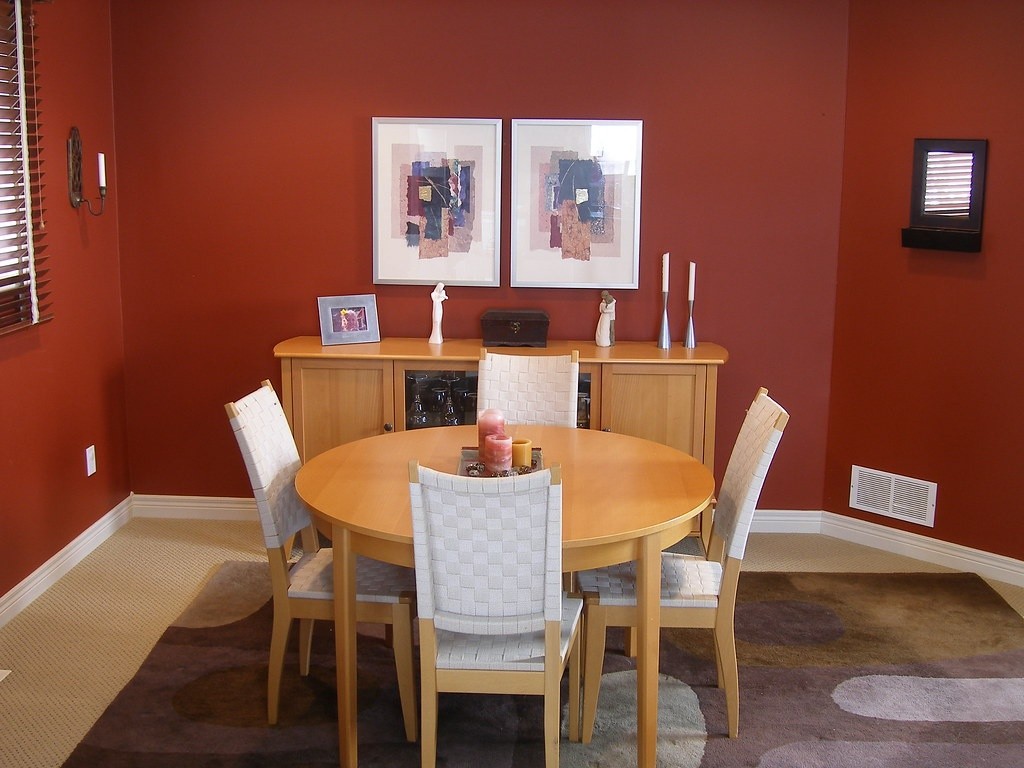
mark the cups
[405,372,476,429]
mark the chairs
[575,386,789,746]
[406,457,586,767]
[228,379,419,746]
[477,345,580,431]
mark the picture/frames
[510,120,646,289]
[371,117,502,288]
[316,294,380,345]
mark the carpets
[60,557,1024,768]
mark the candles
[662,252,669,292]
[689,261,696,301]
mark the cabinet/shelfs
[273,335,729,552]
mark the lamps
[69,128,107,215]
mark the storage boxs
[480,308,549,348]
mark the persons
[341,310,358,332]
[428,282,448,344]
[595,291,616,347]
